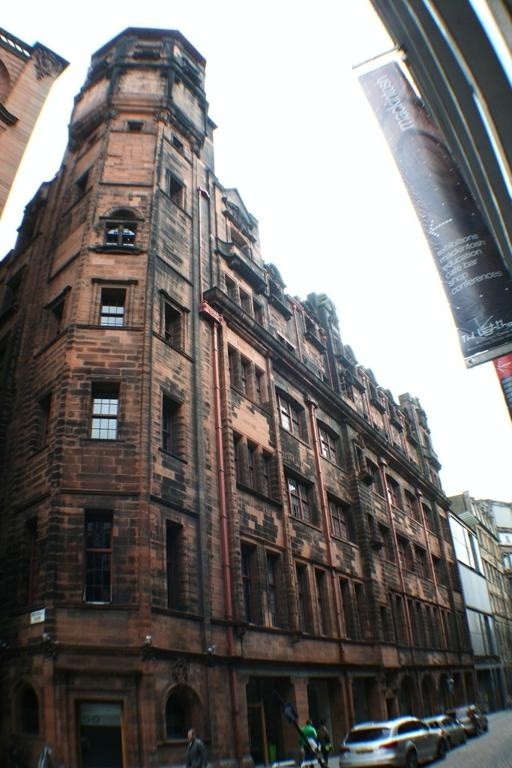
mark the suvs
[338,714,447,768]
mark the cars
[422,714,468,751]
[446,703,489,737]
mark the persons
[185,727,207,768]
[299,719,316,767]
[39,743,55,768]
[314,727,333,767]
